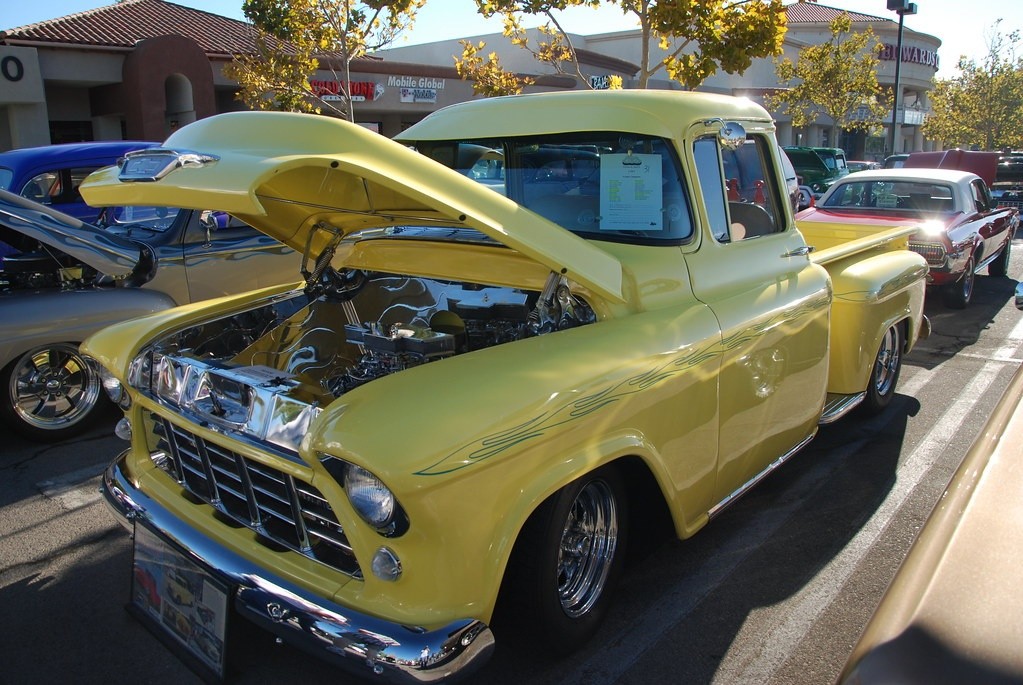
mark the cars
[794,149,1021,309]
[989,151,1023,230]
[458,143,611,200]
[781,145,910,210]
[0,141,232,276]
[1,187,396,440]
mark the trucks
[79,87,932,684]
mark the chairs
[660,199,772,238]
[529,193,618,233]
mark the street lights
[887,0,918,154]
